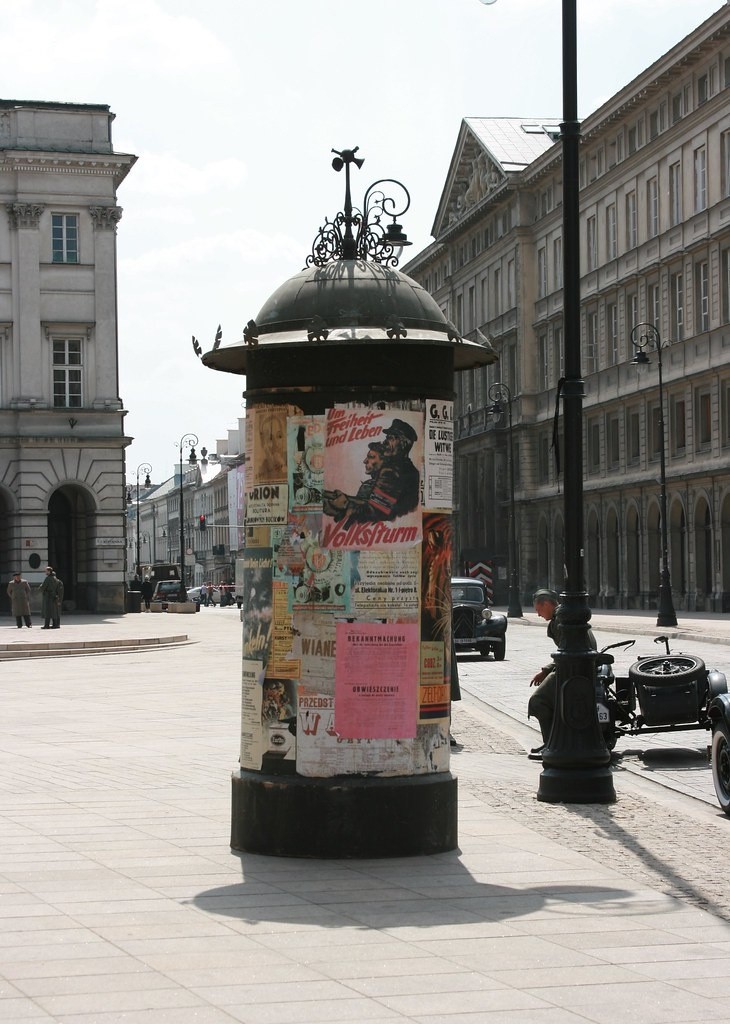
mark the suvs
[153,580,188,608]
[218,585,237,600]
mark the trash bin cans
[128,591,141,613]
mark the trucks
[135,561,183,596]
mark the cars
[186,586,221,604]
[451,578,507,661]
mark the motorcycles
[706,691,730,814]
[594,637,729,756]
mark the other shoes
[41,626,50,629]
[449,734,457,747]
[18,626,22,628]
[532,744,545,753]
[528,748,546,760]
[28,624,32,628]
[145,609,151,613]
[50,626,60,629]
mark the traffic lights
[200,514,207,531]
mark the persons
[198,581,216,607]
[37,566,64,629]
[7,572,32,628]
[528,590,597,761]
[218,581,231,606]
[130,574,153,613]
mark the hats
[13,573,21,576]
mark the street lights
[630,323,680,627]
[175,432,199,602]
[135,463,154,578]
[488,382,524,619]
